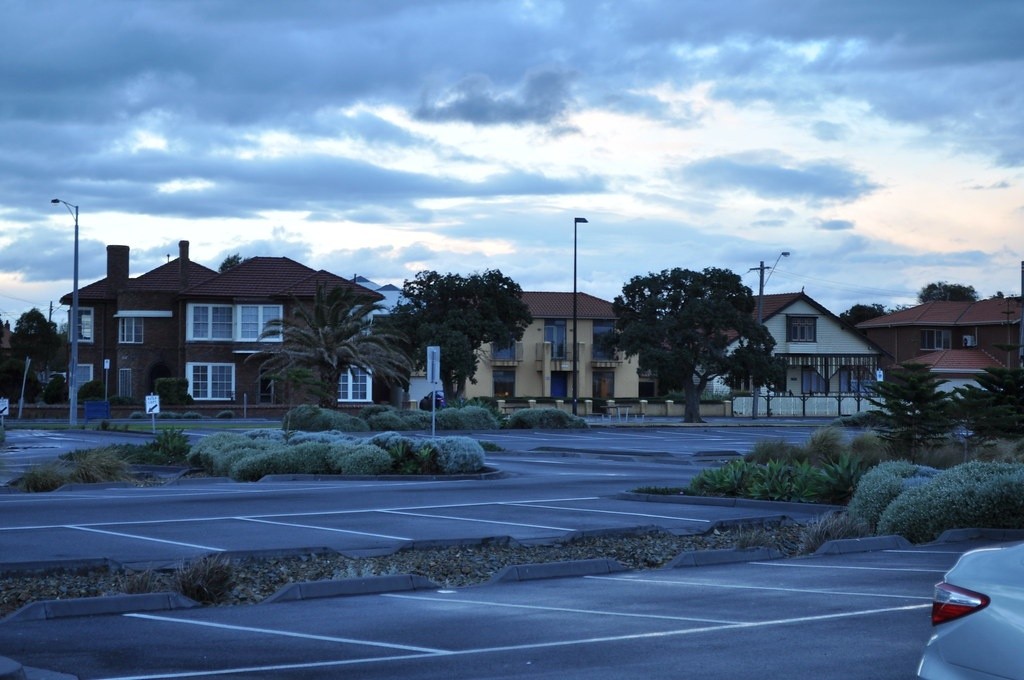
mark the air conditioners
[962,336,975,346]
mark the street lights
[569,217,589,416]
[50,198,81,425]
[752,251,790,419]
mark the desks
[600,405,633,421]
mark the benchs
[588,413,611,422]
[619,413,645,420]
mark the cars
[419,391,466,412]
[916,538,1024,680]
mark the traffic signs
[146,395,160,414]
[0,399,10,416]
